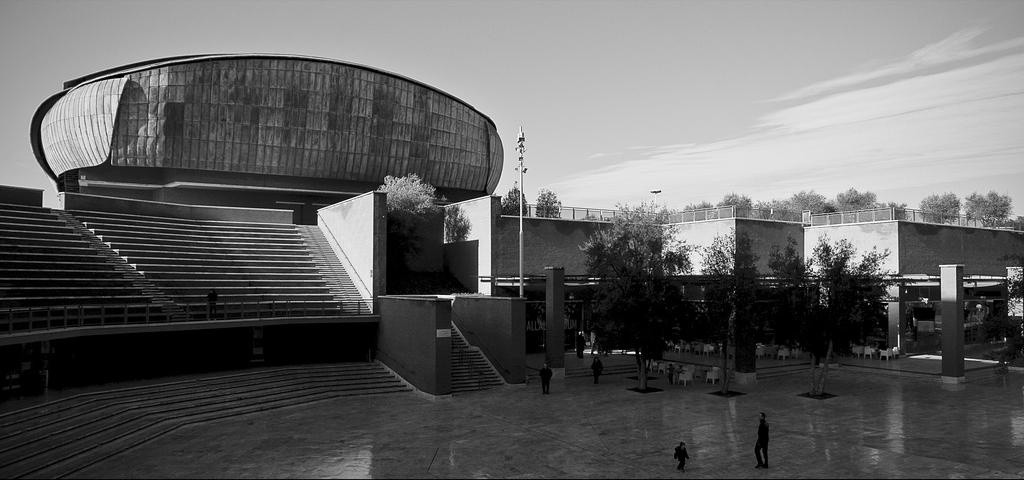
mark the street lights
[651,190,661,201]
[515,125,527,298]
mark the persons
[539,361,552,394]
[575,332,585,358]
[668,363,683,385]
[673,441,689,470]
[754,412,770,469]
[591,356,603,384]
[206,289,218,320]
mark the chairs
[651,359,720,387]
[665,339,802,361]
[852,343,900,360]
